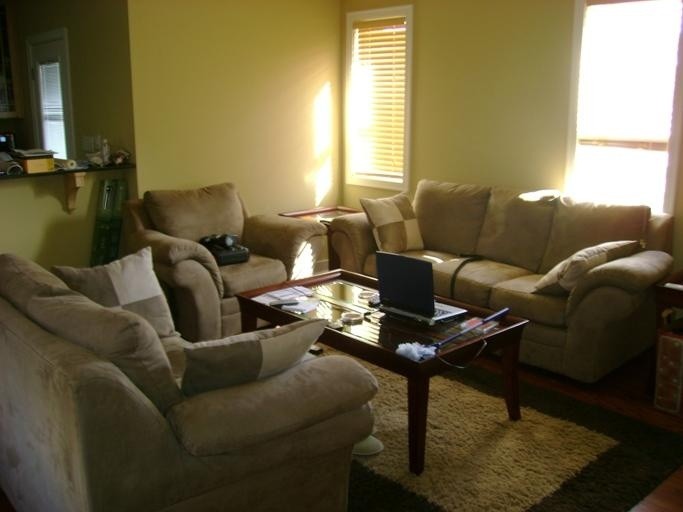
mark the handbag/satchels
[199,233,249,266]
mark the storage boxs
[12,152,53,175]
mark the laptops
[376,250,467,326]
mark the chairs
[124,182,328,343]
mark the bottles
[100,138,112,166]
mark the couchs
[0,244,378,512]
[327,179,675,384]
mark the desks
[277,205,363,229]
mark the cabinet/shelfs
[653,275,682,414]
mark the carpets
[306,341,683,511]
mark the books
[12,146,60,157]
[250,283,321,315]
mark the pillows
[528,239,640,296]
[360,191,425,253]
[51,244,178,340]
[174,314,327,395]
